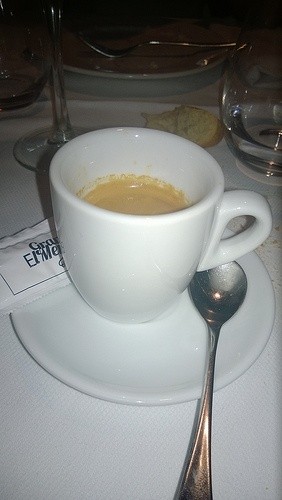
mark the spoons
[177,260,247,500]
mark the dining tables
[0,21,282,500]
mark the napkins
[0,211,72,315]
[238,27,282,90]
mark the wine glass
[14,1,93,173]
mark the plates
[59,15,233,78]
[9,227,276,407]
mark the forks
[75,30,238,57]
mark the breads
[141,105,224,147]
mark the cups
[48,126,273,325]
[0,0,54,113]
[218,0,282,186]
